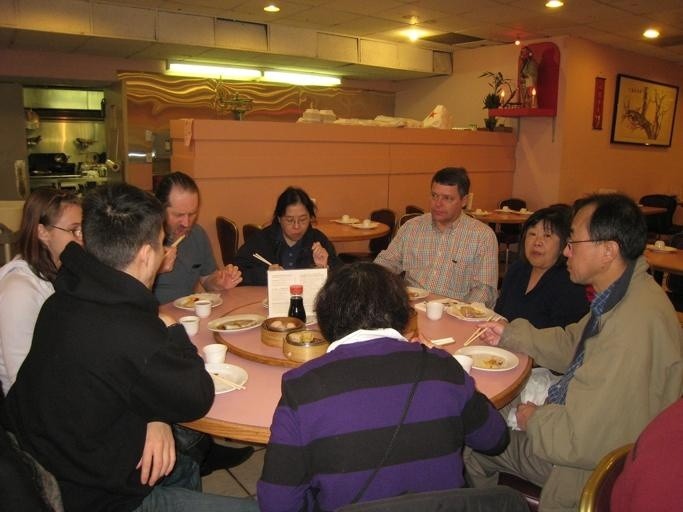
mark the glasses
[565,237,609,251]
[49,225,83,242]
[277,216,309,225]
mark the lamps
[162,57,342,88]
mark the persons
[461,193,683,512]
[493,207,590,331]
[371,167,499,310]
[154,171,245,305]
[234,187,339,285]
[256,261,511,511]
[609,395,683,512]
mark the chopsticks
[162,234,185,258]
[211,374,245,390]
[431,337,454,346]
[463,315,501,347]
[252,252,271,266]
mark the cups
[503,206,507,211]
[342,215,349,222]
[654,240,665,249]
[452,354,473,373]
[519,209,526,214]
[476,209,481,215]
[178,316,199,338]
[202,343,227,363]
[363,219,370,228]
[425,301,443,321]
[194,301,211,319]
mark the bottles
[288,285,305,323]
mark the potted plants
[477,70,513,131]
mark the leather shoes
[199,443,255,478]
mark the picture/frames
[610,74,678,148]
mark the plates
[471,212,492,217]
[203,363,248,394]
[206,313,266,332]
[646,246,676,254]
[352,224,377,230]
[447,304,494,322]
[407,287,428,300]
[495,210,514,214]
[335,219,358,224]
[454,345,520,371]
[514,212,534,215]
[173,292,222,311]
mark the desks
[636,241,682,310]
[465,208,536,283]
[259,215,389,257]
[633,203,666,238]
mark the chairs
[214,216,241,267]
[323,484,530,512]
[581,444,633,512]
[398,214,422,228]
[499,198,526,270]
[0,426,70,512]
[337,208,395,262]
[243,223,261,247]
[641,193,676,241]
[407,205,425,218]
